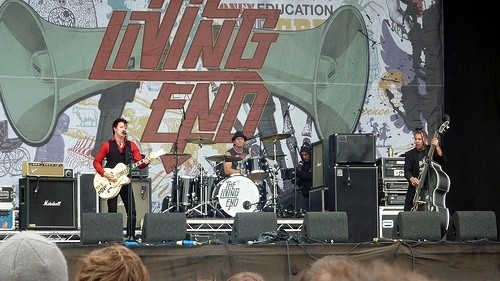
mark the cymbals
[260,132,291,143]
[164,152,191,157]
[183,137,217,144]
[266,153,287,158]
[207,154,242,162]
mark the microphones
[182,106,187,120]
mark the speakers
[302,212,349,243]
[81,213,123,243]
[447,211,497,241]
[234,212,276,242]
[395,212,441,241]
[142,213,187,241]
[20,177,77,230]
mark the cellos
[411,112,451,241]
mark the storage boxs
[0,202,16,231]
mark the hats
[231,131,247,142]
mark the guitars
[93,148,167,199]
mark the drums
[171,176,200,208]
[244,155,270,180]
[211,173,260,217]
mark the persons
[0,230,71,281]
[291,141,314,212]
[402,129,446,210]
[217,131,252,177]
[73,241,153,281]
[221,254,429,281]
[94,118,151,242]
